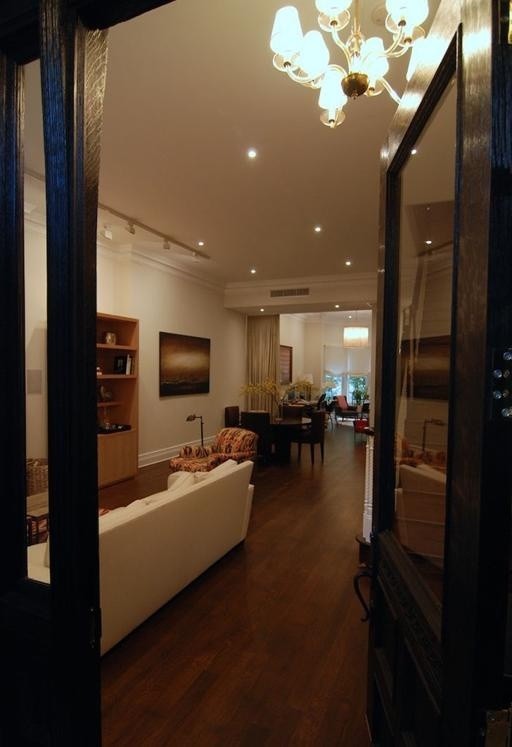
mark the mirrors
[393,71,445,608]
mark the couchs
[26,459,254,659]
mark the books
[113,354,133,374]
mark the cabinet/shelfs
[97,313,139,487]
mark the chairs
[396,463,446,562]
[225,405,325,469]
[169,427,259,479]
[333,395,358,422]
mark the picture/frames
[280,345,292,384]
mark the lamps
[270,0,428,129]
[344,310,369,348]
